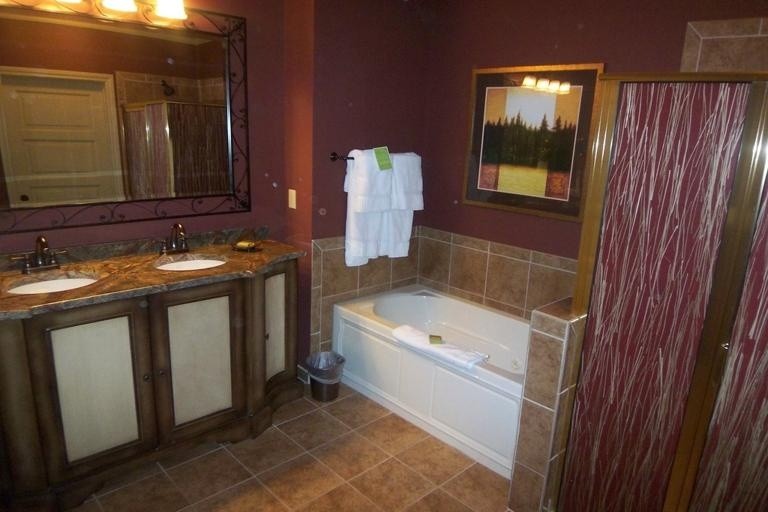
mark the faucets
[34,235,48,266]
[169,223,186,249]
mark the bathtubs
[330,282,531,481]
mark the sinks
[7,271,109,296]
[154,253,228,271]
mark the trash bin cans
[306,351,345,402]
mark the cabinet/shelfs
[249,258,304,440]
[0,280,250,512]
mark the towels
[391,322,490,370]
[344,148,424,268]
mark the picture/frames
[461,62,606,224]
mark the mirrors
[0,0,252,234]
